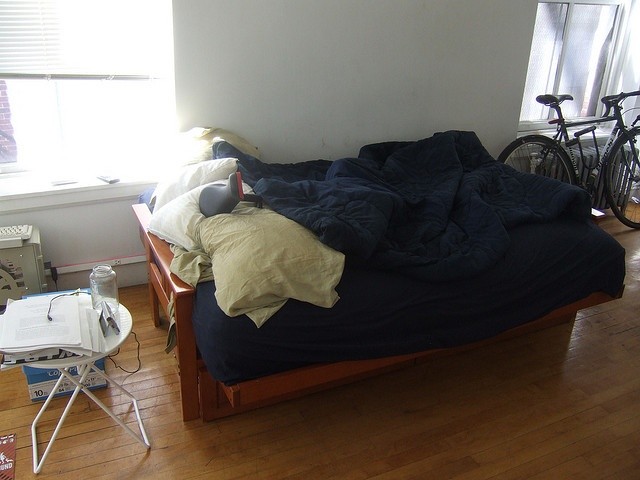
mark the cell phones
[99,300,120,338]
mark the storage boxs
[20,287,107,403]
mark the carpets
[0,432,16,480]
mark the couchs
[132,198,624,422]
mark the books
[0,288,107,357]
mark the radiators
[530,144,639,217]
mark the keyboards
[0,225,33,240]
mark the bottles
[89,265,119,315]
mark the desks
[6,292,151,475]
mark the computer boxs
[0,228,47,305]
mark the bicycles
[497,86,640,230]
